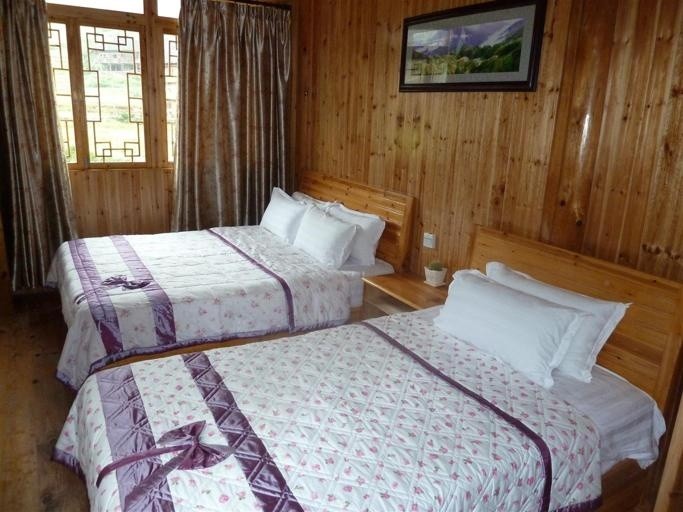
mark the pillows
[433,269,587,392]
[485,261,634,385]
[259,186,307,245]
[325,200,386,265]
[294,203,362,271]
[292,191,334,212]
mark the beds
[46,223,683,512]
[45,169,414,393]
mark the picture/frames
[399,0,548,93]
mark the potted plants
[423,260,448,288]
